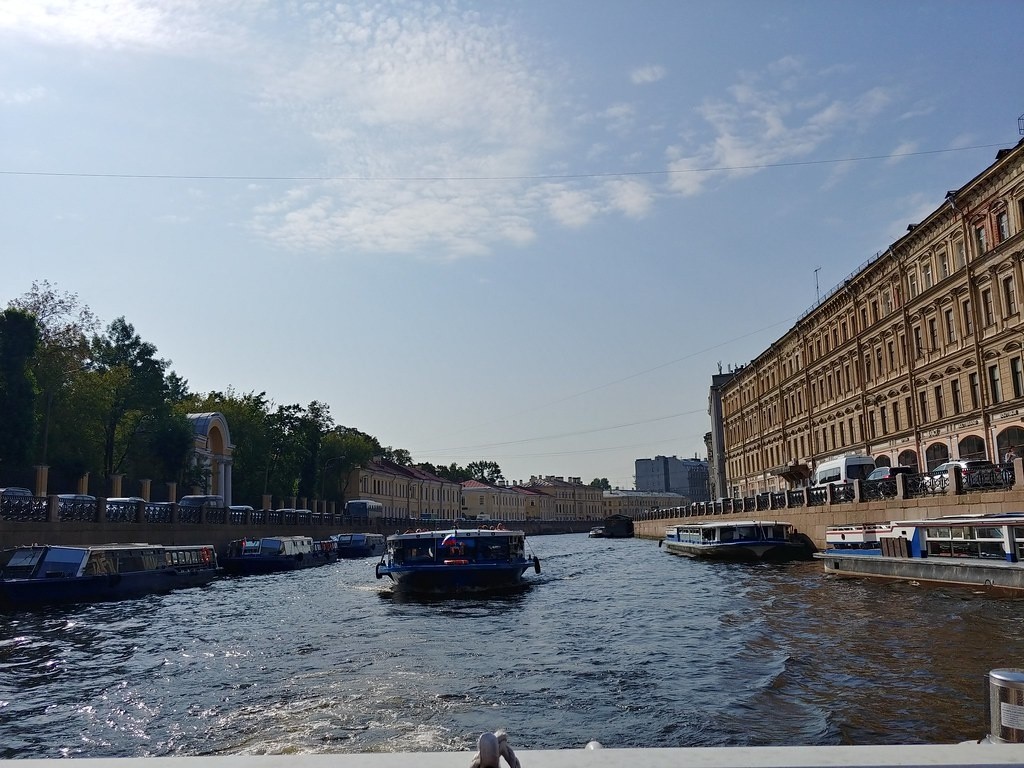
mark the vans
[180,493,224,509]
[811,455,876,498]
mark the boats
[226,534,338,573]
[374,521,540,592]
[328,530,385,558]
[812,511,1024,597]
[655,519,804,561]
[0,543,225,599]
[588,515,635,537]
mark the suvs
[862,465,917,498]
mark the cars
[107,498,144,515]
[228,504,254,522]
[919,458,1004,495]
[145,501,180,518]
[642,485,812,519]
[256,507,342,525]
[0,487,36,505]
[42,494,97,511]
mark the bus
[345,500,382,525]
